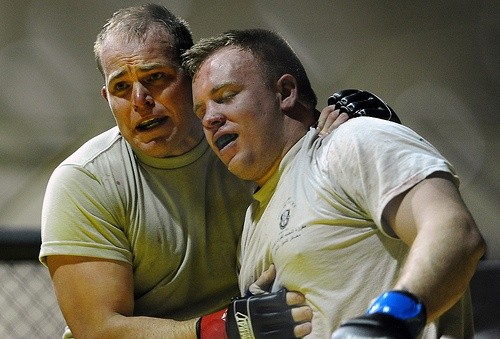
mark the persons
[181,29,486,339]
[39,3,401,339]
